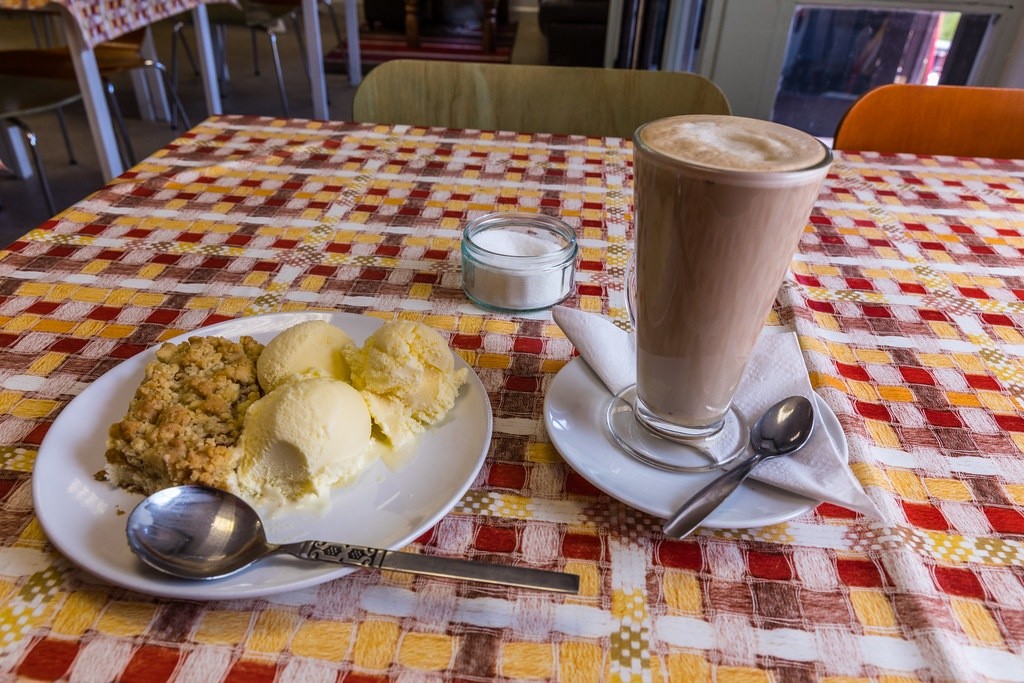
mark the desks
[0,116,1024,683]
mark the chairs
[832,84,1024,160]
[169,0,343,129]
[351,60,731,140]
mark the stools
[0,48,139,217]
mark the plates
[543,354,847,528]
[33,311,494,601]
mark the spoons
[662,395,814,540]
[127,484,580,594]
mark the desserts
[256,320,353,388]
[344,321,467,446]
[241,378,371,498]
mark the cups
[608,113,836,472]
[462,211,580,313]
